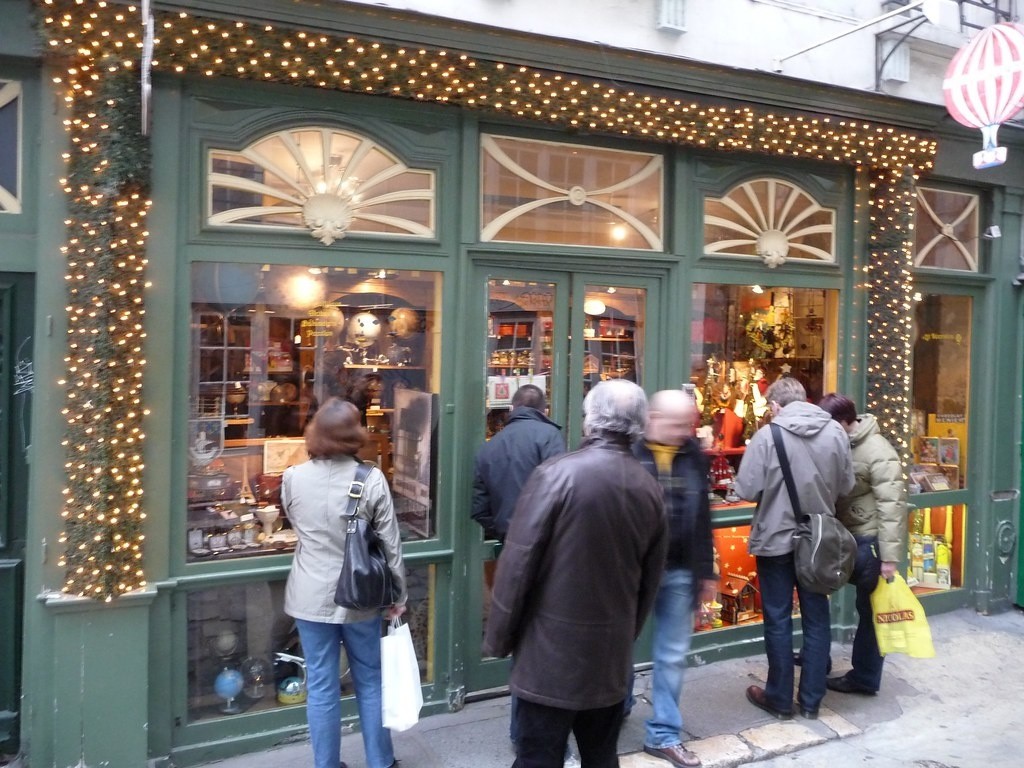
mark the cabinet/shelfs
[241,370,298,407]
[793,314,825,360]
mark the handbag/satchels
[333,461,399,609]
[795,509,858,595]
[870,571,937,659]
[380,615,424,732]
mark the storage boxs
[599,320,630,337]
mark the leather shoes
[643,743,702,768]
[793,652,832,674]
[825,670,875,696]
[799,706,818,719]
[747,685,791,719]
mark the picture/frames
[908,437,959,490]
[264,440,312,475]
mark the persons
[280,395,408,768]
[471,377,720,768]
[735,376,908,719]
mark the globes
[213,669,245,715]
